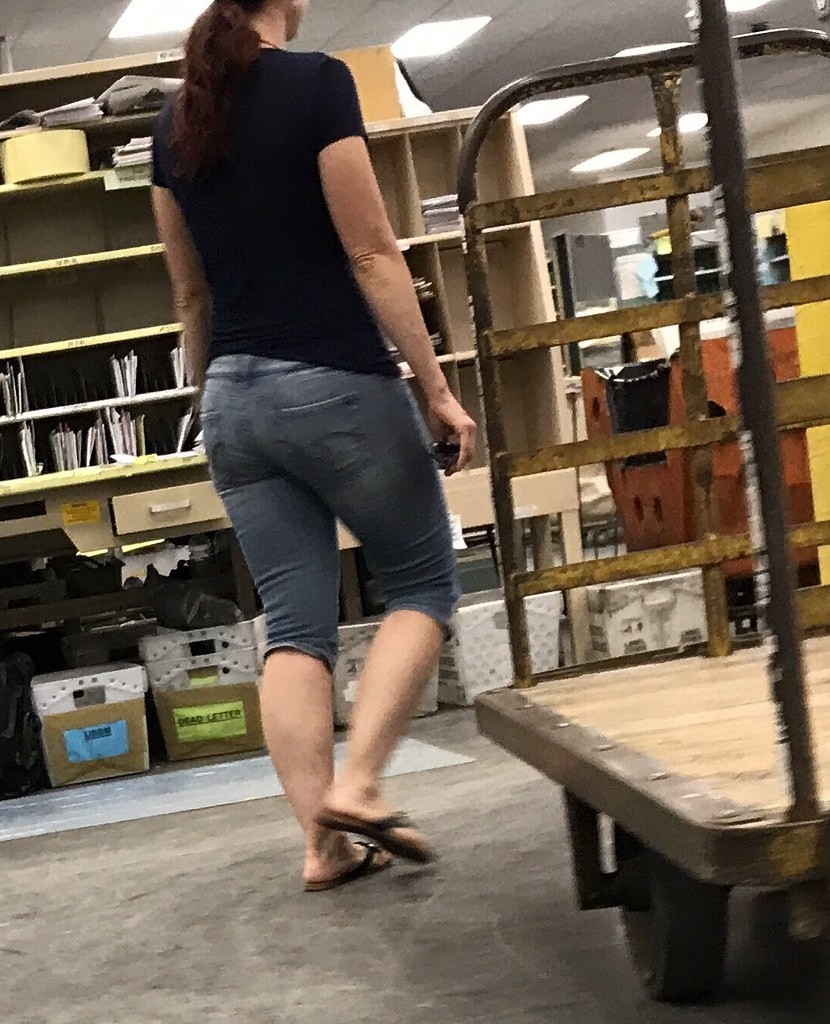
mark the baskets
[30,661,148,788]
[438,587,560,707]
[331,614,439,725]
[137,613,269,761]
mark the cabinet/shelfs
[368,102,575,449]
[0,45,208,496]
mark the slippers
[315,808,431,863]
[304,842,392,890]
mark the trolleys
[455,27,829,1006]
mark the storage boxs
[331,42,403,122]
[43,697,150,788]
[584,568,708,660]
[333,620,439,725]
[435,589,563,707]
[154,682,267,759]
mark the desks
[584,321,820,595]
[337,467,595,667]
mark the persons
[150,1,478,893]
